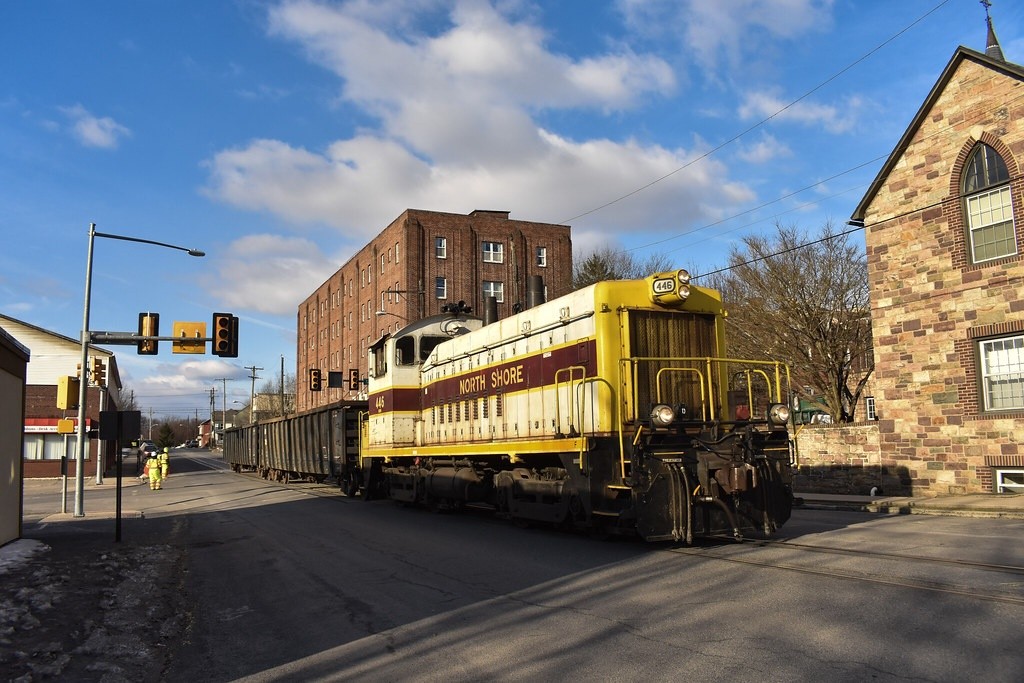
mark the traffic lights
[348,368,359,391]
[91,356,106,389]
[212,310,231,354]
[311,369,321,391]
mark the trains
[222,270,793,548]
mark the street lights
[233,400,253,424]
[150,424,158,440]
[74,221,205,518]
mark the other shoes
[152,487,156,489]
[156,488,163,489]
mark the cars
[143,445,159,459]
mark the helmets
[164,447,168,452]
[151,451,156,456]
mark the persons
[140,447,169,490]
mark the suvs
[189,441,198,448]
